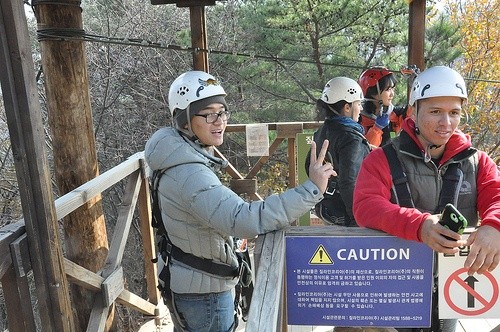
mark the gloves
[375,113,390,130]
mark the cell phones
[438,203,467,249]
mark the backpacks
[315,187,350,226]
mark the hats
[174,95,229,129]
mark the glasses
[195,111,231,124]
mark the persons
[351,66,500,276]
[144,70,338,332]
[357,66,416,152]
[305,76,373,227]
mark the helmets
[358,66,393,97]
[321,77,364,105]
[409,66,468,106]
[168,70,227,125]
